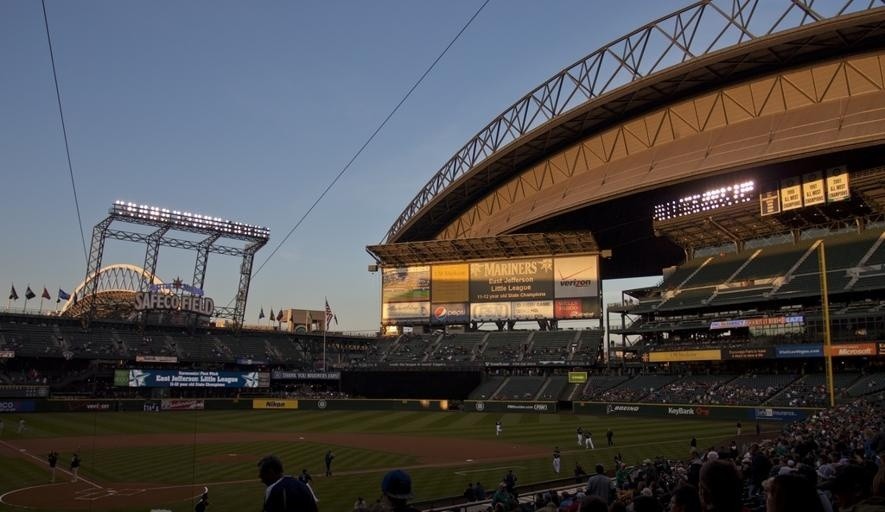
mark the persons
[1,318,885,512]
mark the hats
[816,461,872,491]
[379,468,417,501]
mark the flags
[9,284,19,300]
[43,287,50,299]
[259,309,283,320]
[326,301,332,330]
[25,286,35,300]
[60,289,70,301]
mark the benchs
[618,223,884,317]
